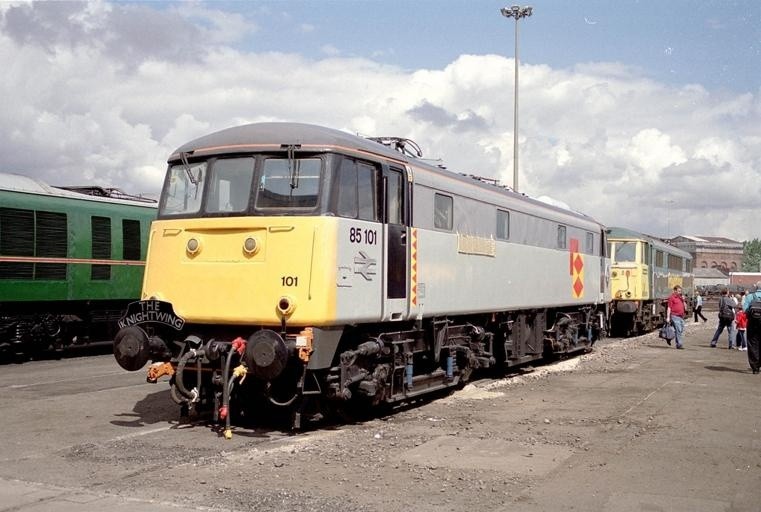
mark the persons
[711,280,761,374]
[665,285,688,349]
[694,291,708,322]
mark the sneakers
[710,343,716,348]
[729,345,747,351]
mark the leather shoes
[666,339,671,346]
[676,344,682,349]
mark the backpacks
[749,292,761,321]
[717,306,734,322]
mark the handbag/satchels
[659,324,675,339]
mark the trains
[112,123,694,442]
[1,170,165,364]
